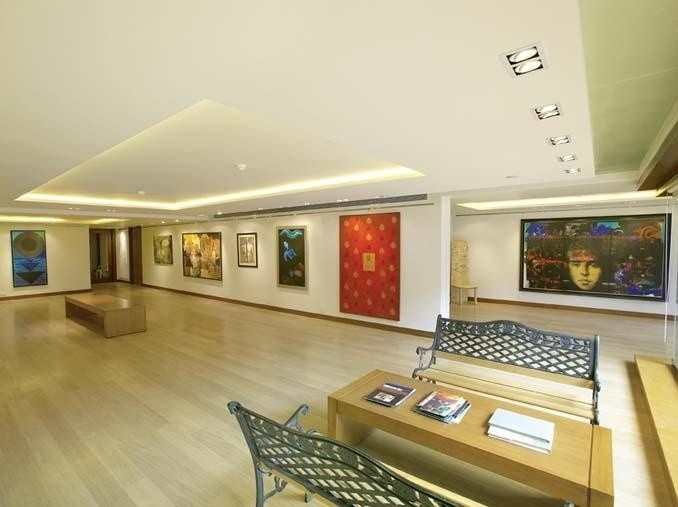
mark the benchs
[227,400,489,506]
[64,293,147,336]
[410,313,602,425]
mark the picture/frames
[518,212,671,302]
[274,224,309,289]
[237,232,258,267]
[181,231,222,281]
[10,230,48,287]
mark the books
[363,380,555,454]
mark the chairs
[96,264,109,279]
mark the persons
[282,239,298,279]
[558,238,615,294]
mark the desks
[452,283,479,304]
